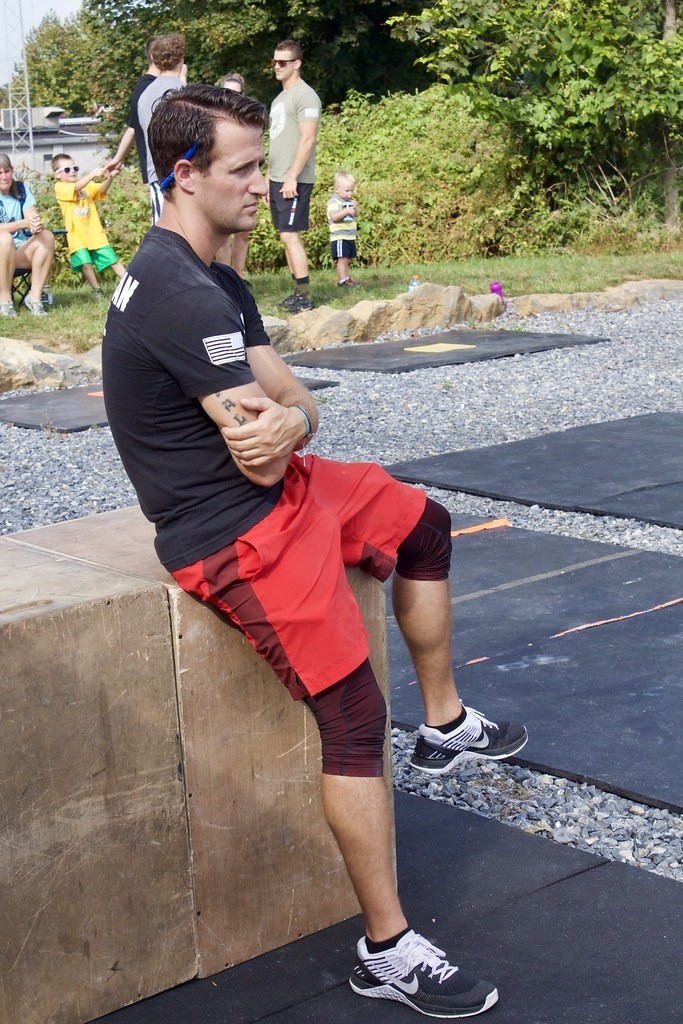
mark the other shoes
[93,288,104,298]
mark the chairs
[10,230,68,308]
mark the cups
[490,281,504,305]
[342,202,355,222]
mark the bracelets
[293,404,313,440]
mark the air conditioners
[0,109,29,130]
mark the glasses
[55,165,79,173]
[271,59,297,67]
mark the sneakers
[338,278,354,287]
[349,929,499,1018]
[24,290,48,316]
[273,291,316,314]
[409,699,528,774]
[0,299,17,317]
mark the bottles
[408,275,420,291]
[41,282,53,305]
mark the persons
[261,40,322,317]
[137,33,187,226]
[326,170,360,290]
[98,36,162,184]
[101,83,528,1018]
[0,153,54,318]
[216,72,249,279]
[51,153,126,300]
